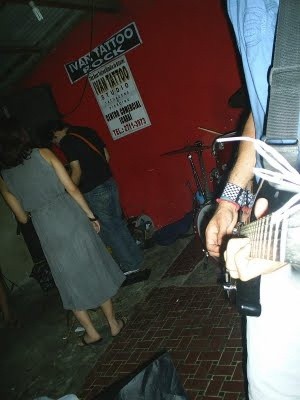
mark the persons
[48,125,144,275]
[205,0,300,400]
[0,282,13,321]
[0,120,129,347]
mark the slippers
[77,336,103,348]
[112,317,128,337]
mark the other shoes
[121,268,151,288]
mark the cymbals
[160,146,212,157]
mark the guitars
[217,178,300,317]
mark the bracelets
[222,182,245,203]
[89,215,98,221]
[215,195,241,210]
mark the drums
[193,199,220,267]
[213,132,241,175]
